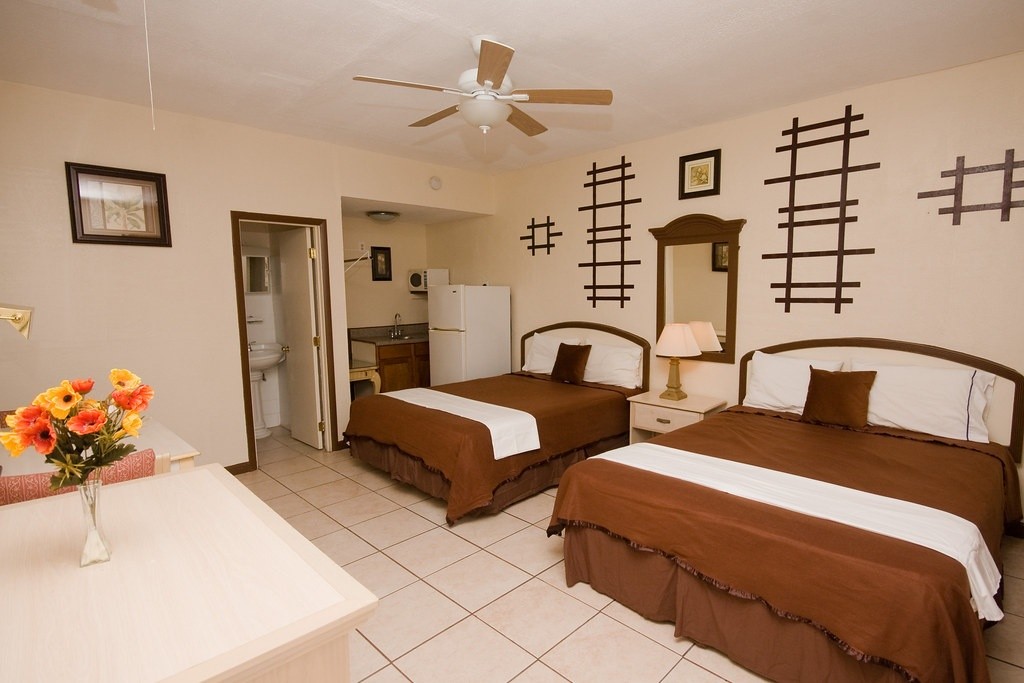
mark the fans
[352,34,614,137]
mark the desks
[0,412,204,478]
[349,359,382,395]
[0,463,377,683]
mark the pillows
[798,364,878,432]
[850,358,996,444]
[740,350,844,415]
[550,342,592,385]
[521,332,582,375]
[582,339,642,389]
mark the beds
[545,337,1024,683]
[342,321,651,529]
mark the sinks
[395,336,414,339]
[248,351,277,358]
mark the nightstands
[627,389,727,445]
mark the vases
[76,479,111,567]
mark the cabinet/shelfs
[376,341,431,394]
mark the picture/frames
[678,148,722,200]
[371,246,393,281]
[712,242,728,272]
[65,161,173,248]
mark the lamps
[652,323,702,402]
[367,211,400,222]
[687,321,723,352]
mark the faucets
[389,313,404,338]
[248,339,256,351]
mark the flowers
[0,368,155,527]
[458,94,513,134]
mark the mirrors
[649,214,747,364]
[242,255,270,294]
